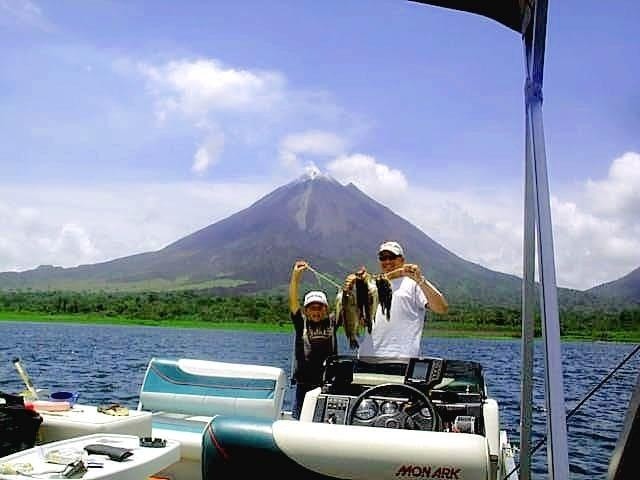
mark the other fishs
[335,266,392,349]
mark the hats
[380,241,406,258]
[304,290,330,304]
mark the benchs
[137,355,288,463]
[201,414,492,479]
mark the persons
[288,260,343,420]
[357,240,448,357]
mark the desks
[39,403,152,441]
[0,433,182,480]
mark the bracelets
[417,276,425,285]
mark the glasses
[379,254,401,261]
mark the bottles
[23,391,34,411]
